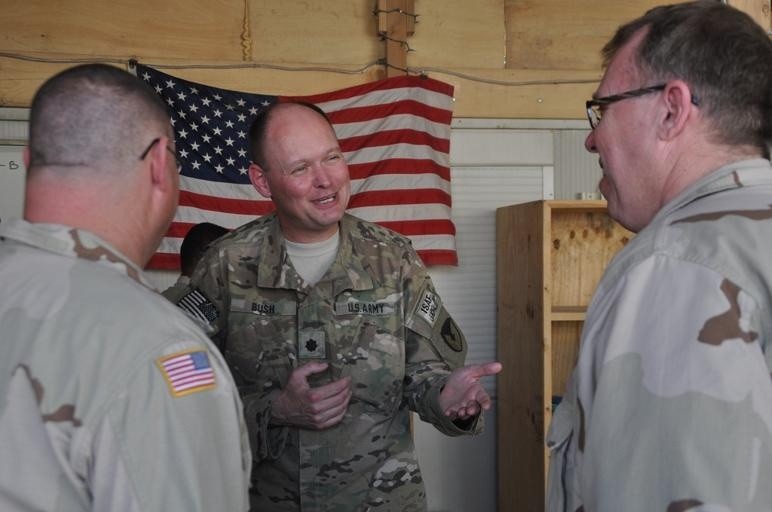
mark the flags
[126,53,461,273]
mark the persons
[170,100,503,512]
[542,0,770,512]
[1,61,255,512]
[157,219,237,305]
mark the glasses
[138,136,186,175]
[583,79,702,130]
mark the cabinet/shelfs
[495,198,640,511]
